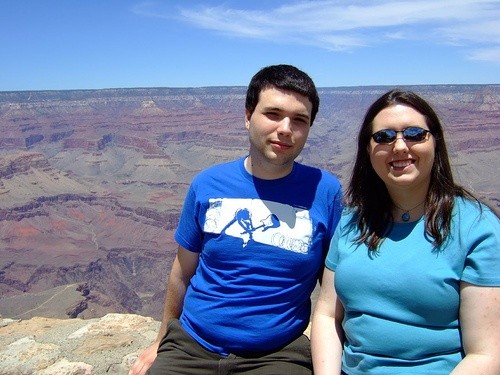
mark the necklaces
[394,199,427,222]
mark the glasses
[370,127,432,144]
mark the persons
[310,89,500,375]
[125,63,345,375]
